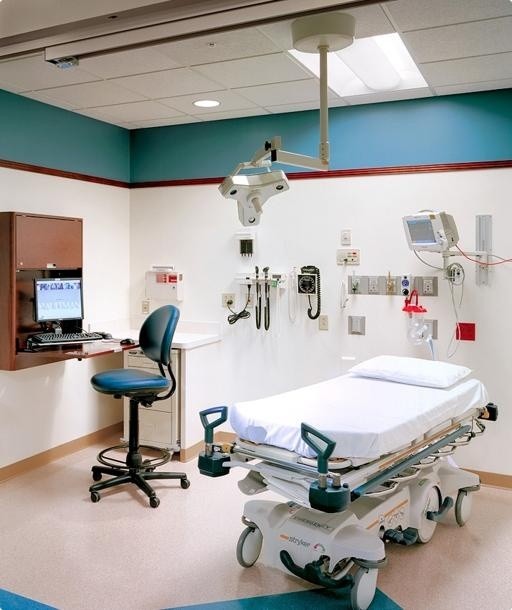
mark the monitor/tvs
[31,277,84,334]
[402,210,459,253]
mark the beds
[196,371,501,608]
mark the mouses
[120,338,135,345]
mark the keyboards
[32,332,103,343]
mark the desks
[120,336,221,464]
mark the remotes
[96,331,113,339]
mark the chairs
[88,304,190,510]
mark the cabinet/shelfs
[0,212,92,372]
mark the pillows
[349,355,473,389]
